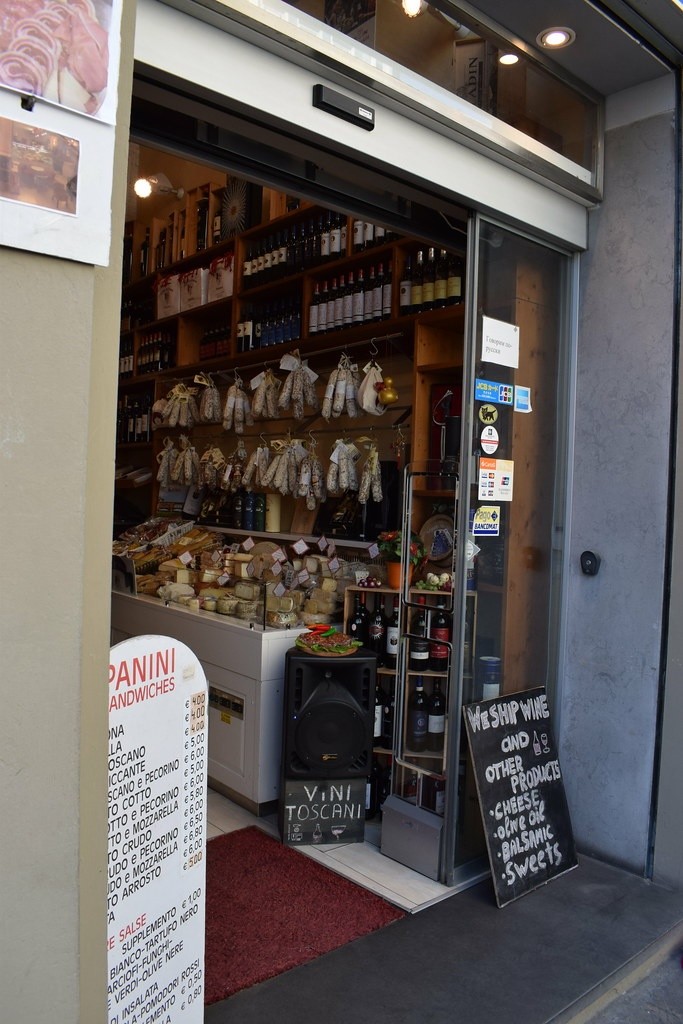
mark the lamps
[402,0,471,39]
[134,176,185,199]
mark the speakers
[281,646,377,779]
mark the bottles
[365,674,445,822]
[117,191,468,444]
[347,591,452,672]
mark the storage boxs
[157,256,234,320]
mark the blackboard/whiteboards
[463,686,580,909]
[285,779,367,845]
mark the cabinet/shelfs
[400,587,478,834]
[113,172,559,835]
[345,585,407,794]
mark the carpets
[204,825,406,1005]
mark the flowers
[377,529,430,576]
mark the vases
[386,561,413,590]
